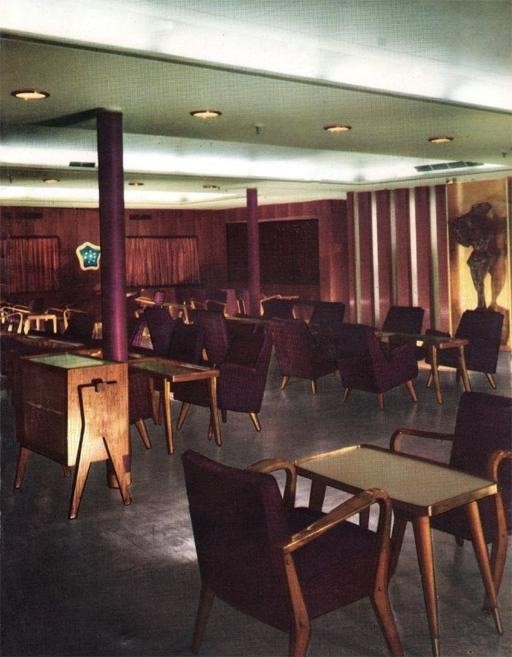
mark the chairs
[181,449,405,655]
[387,391,509,613]
[260,295,504,410]
[1,287,273,455]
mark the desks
[293,444,504,656]
[15,353,131,516]
